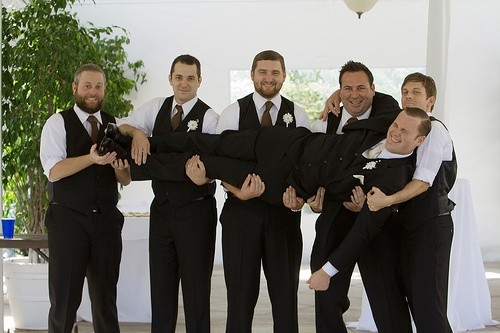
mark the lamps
[344,0,378,19]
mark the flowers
[186,119,199,132]
[283,113,293,128]
[362,160,382,170]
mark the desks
[0,235,78,333]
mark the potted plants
[0,0,148,330]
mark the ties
[87,115,99,143]
[261,101,276,126]
[365,142,385,158]
[171,105,185,129]
[347,117,359,124]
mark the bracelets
[290,209,301,212]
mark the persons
[310,60,414,333]
[114,55,221,333]
[215,50,310,333]
[98,107,431,290]
[39,63,135,333]
[319,73,457,333]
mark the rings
[187,165,191,167]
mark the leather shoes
[106,121,132,150]
[99,137,127,157]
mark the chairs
[78,180,155,322]
[346,178,500,333]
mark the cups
[1,218,16,238]
